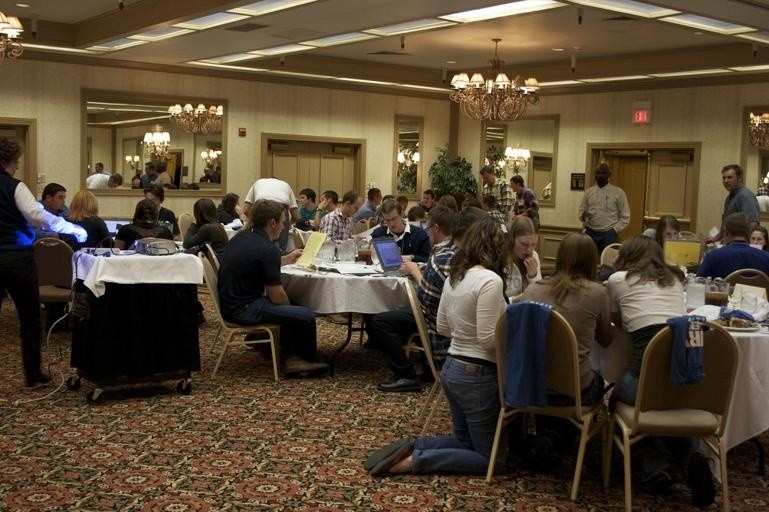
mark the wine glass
[728,286,742,311]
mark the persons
[480,166,515,214]
[578,164,630,254]
[31,182,244,259]
[87,162,221,190]
[0,133,87,389]
[510,175,541,230]
[365,214,769,507]
[703,164,760,244]
[217,177,504,391]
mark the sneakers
[693,459,717,508]
[365,437,406,469]
[371,442,413,478]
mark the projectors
[135,236,176,255]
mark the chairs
[484,301,603,502]
[400,331,451,362]
[197,251,284,382]
[36,238,81,339]
[177,212,376,275]
[403,276,446,443]
[599,242,624,266]
[603,318,741,511]
[724,267,767,289]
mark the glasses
[424,224,432,232]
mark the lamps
[200,147,222,169]
[446,39,542,124]
[501,141,530,173]
[745,108,768,149]
[124,151,140,170]
[167,101,224,137]
[140,117,172,155]
[0,13,30,61]
[397,142,420,169]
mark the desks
[677,311,769,460]
[278,257,409,377]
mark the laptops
[103,218,132,238]
[373,236,425,276]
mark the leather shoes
[284,356,330,378]
[379,376,420,393]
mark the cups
[740,295,757,316]
[356,238,372,265]
[695,276,727,293]
[339,239,354,261]
[401,255,411,264]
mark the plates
[712,320,761,331]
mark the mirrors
[478,113,560,209]
[738,104,768,224]
[392,112,425,202]
[78,87,230,199]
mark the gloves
[67,224,88,242]
